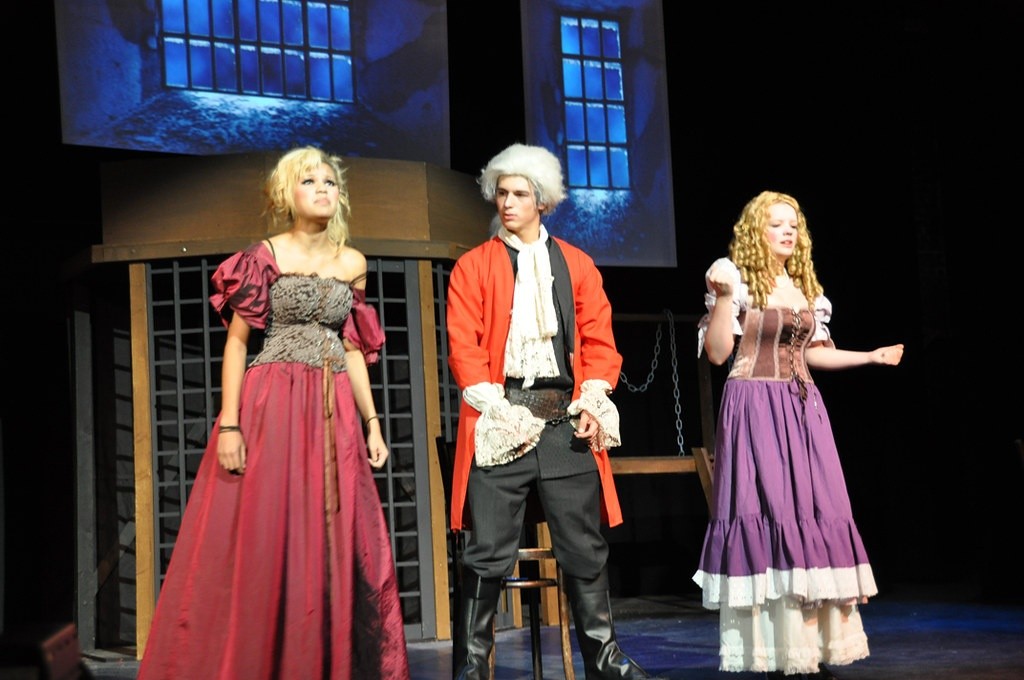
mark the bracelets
[219,426,242,433]
[365,416,379,428]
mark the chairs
[436,435,576,680]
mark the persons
[697,190,904,678]
[449,142,652,680]
[137,146,410,680]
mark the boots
[563,564,654,680]
[452,568,503,680]
